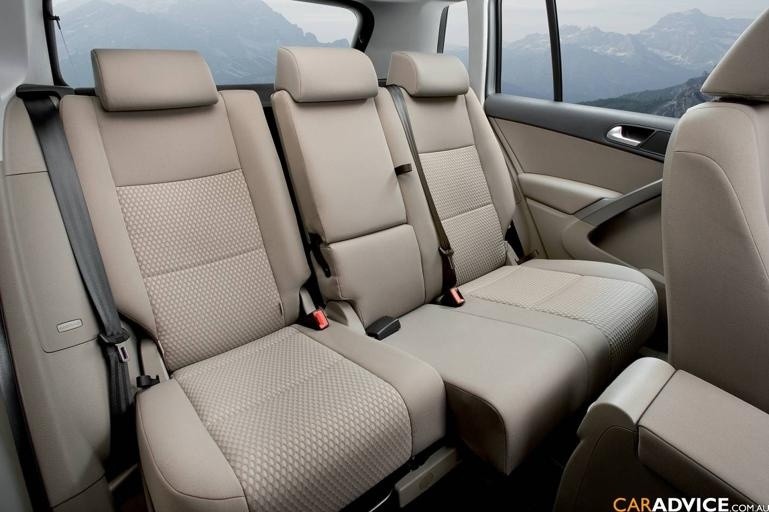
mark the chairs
[61,8,769,512]
